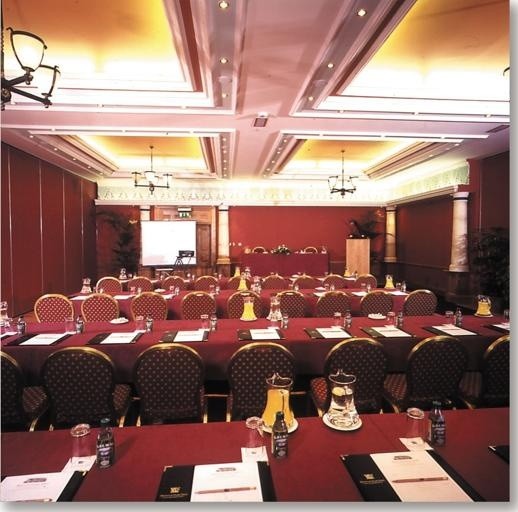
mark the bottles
[175,285,179,296]
[96,419,114,470]
[344,310,352,329]
[456,306,462,326]
[77,316,84,332]
[396,282,400,288]
[401,280,406,293]
[137,288,141,294]
[5,322,11,333]
[271,412,289,458]
[17,315,25,335]
[210,312,217,331]
[367,282,371,292]
[0,320,6,345]
[397,310,403,329]
[428,401,446,446]
[215,287,220,295]
[283,311,288,329]
[147,313,154,332]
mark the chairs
[292,275,317,290]
[403,289,437,316]
[81,293,120,322]
[227,290,263,320]
[314,291,353,318]
[359,290,394,317]
[280,290,308,319]
[307,337,388,416]
[0,349,49,433]
[260,274,287,289]
[161,275,187,292]
[34,293,75,323]
[95,276,123,294]
[351,273,378,289]
[180,291,216,321]
[461,337,510,407]
[382,334,468,413]
[321,274,347,289]
[130,342,208,427]
[194,275,217,291]
[129,292,168,320]
[303,245,318,253]
[126,276,154,292]
[40,345,130,430]
[226,341,297,423]
[226,276,251,291]
[251,246,266,254]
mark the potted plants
[460,223,510,313]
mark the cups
[69,423,93,467]
[332,312,341,330]
[384,274,396,290]
[241,296,257,320]
[130,286,136,294]
[288,283,294,290]
[445,311,455,328]
[405,408,426,450]
[503,310,509,325]
[135,313,145,334]
[386,312,397,330]
[63,315,76,334]
[233,266,241,278]
[200,314,209,331]
[209,284,214,292]
[396,283,401,291]
[360,282,366,292]
[267,296,283,320]
[237,275,249,291]
[81,278,92,294]
[0,300,11,328]
[261,372,295,429]
[244,417,265,461]
[327,368,360,429]
[170,285,174,294]
[269,313,279,329]
[324,283,329,292]
[476,294,492,316]
[343,265,351,277]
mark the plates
[322,413,364,432]
[473,313,494,318]
[259,418,299,434]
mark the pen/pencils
[442,329,460,330]
[110,335,134,338]
[392,477,448,483]
[178,333,202,336]
[254,332,274,334]
[15,499,51,502]
[194,487,256,494]
[379,330,398,332]
[35,336,54,339]
[322,330,341,332]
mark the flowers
[269,243,293,256]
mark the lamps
[0,18,65,112]
[130,173,173,197]
[327,172,360,198]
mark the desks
[240,254,329,279]
[67,289,410,316]
[0,404,509,503]
[0,313,511,380]
[119,276,357,291]
